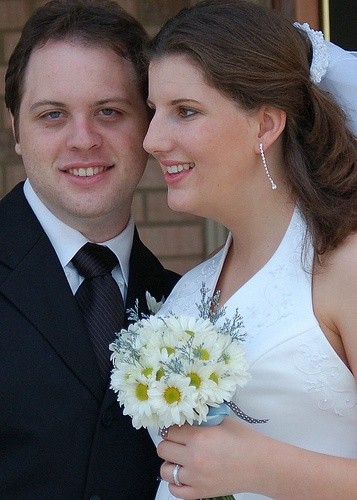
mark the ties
[70,243,126,392]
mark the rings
[172,465,182,487]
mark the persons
[0,2,185,500]
[143,3,355,500]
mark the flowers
[108,282,270,500]
